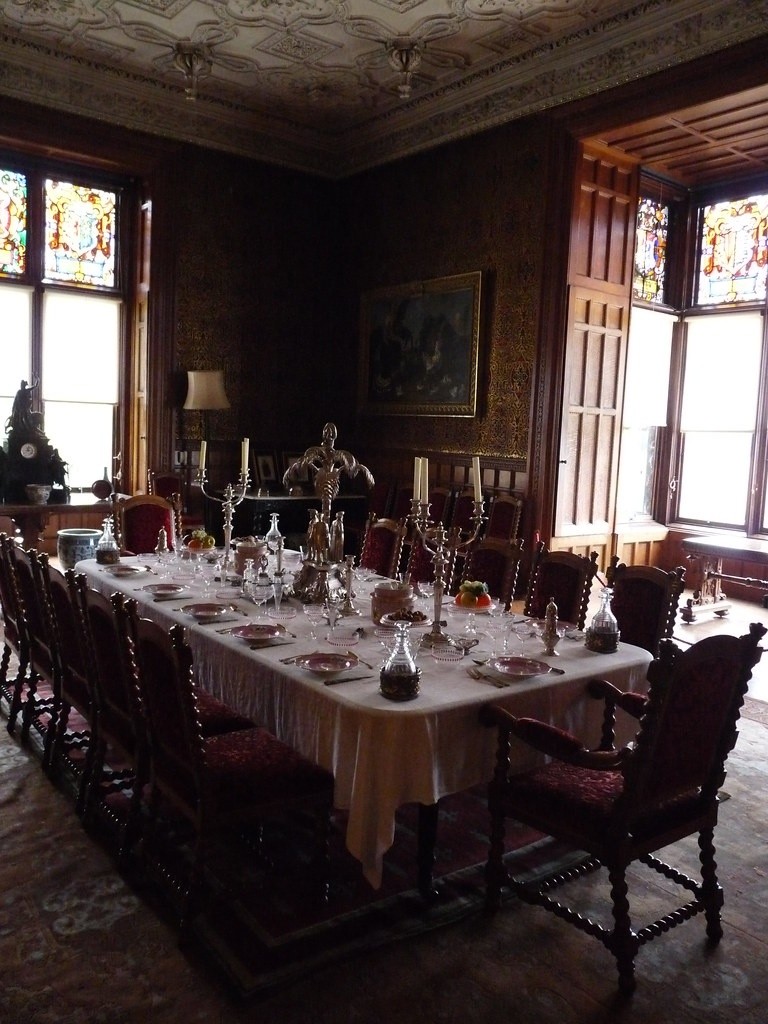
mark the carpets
[0,650,730,1014]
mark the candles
[413,456,421,500]
[421,456,429,504]
[472,457,482,502]
[243,438,249,474]
[241,441,245,474]
[199,441,207,469]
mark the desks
[210,486,368,549]
[45,544,656,904]
[0,492,133,555]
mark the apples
[182,534,215,548]
[455,591,491,606]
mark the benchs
[678,534,768,624]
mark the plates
[104,564,139,578]
[181,602,227,619]
[293,651,358,676]
[142,583,184,597]
[229,624,287,643]
[488,657,551,678]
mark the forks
[465,667,511,688]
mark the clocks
[20,442,38,460]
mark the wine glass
[151,536,527,671]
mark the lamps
[183,369,231,536]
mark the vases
[56,528,103,570]
[25,483,52,505]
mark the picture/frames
[282,450,315,487]
[251,448,281,489]
[355,269,485,418]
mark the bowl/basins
[56,528,102,569]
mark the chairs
[0,469,768,1000]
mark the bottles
[584,586,620,653]
[378,619,421,700]
[95,513,121,564]
